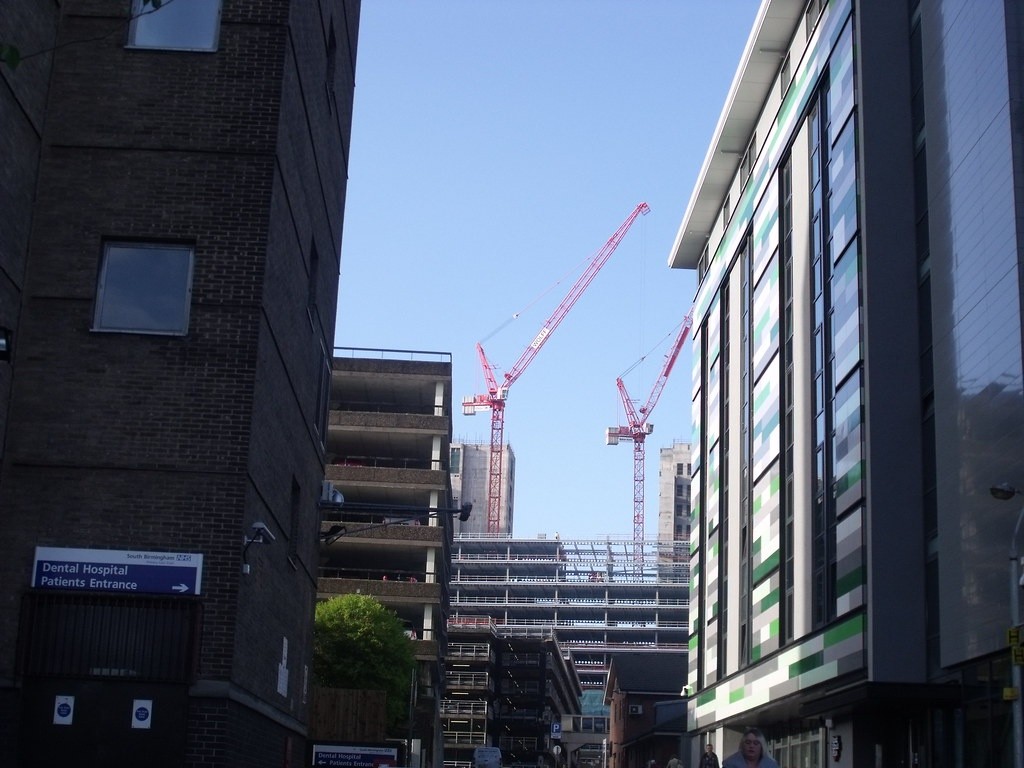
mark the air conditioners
[629,705,642,714]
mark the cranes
[461,202,650,533]
[606,306,694,583]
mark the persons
[666,754,685,768]
[699,744,719,768]
[722,729,779,768]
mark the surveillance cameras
[244,522,276,546]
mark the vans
[471,746,503,768]
